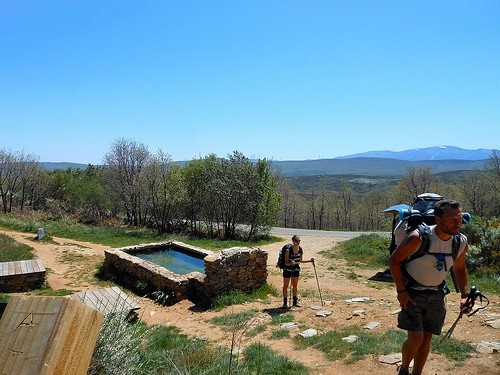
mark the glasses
[294,239,300,242]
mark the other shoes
[283,304,291,309]
[397,365,409,375]
[292,303,302,307]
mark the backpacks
[382,192,471,265]
[276,244,303,269]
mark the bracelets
[397,289,406,293]
[461,292,469,298]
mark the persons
[283,235,315,308]
[388,201,472,375]
